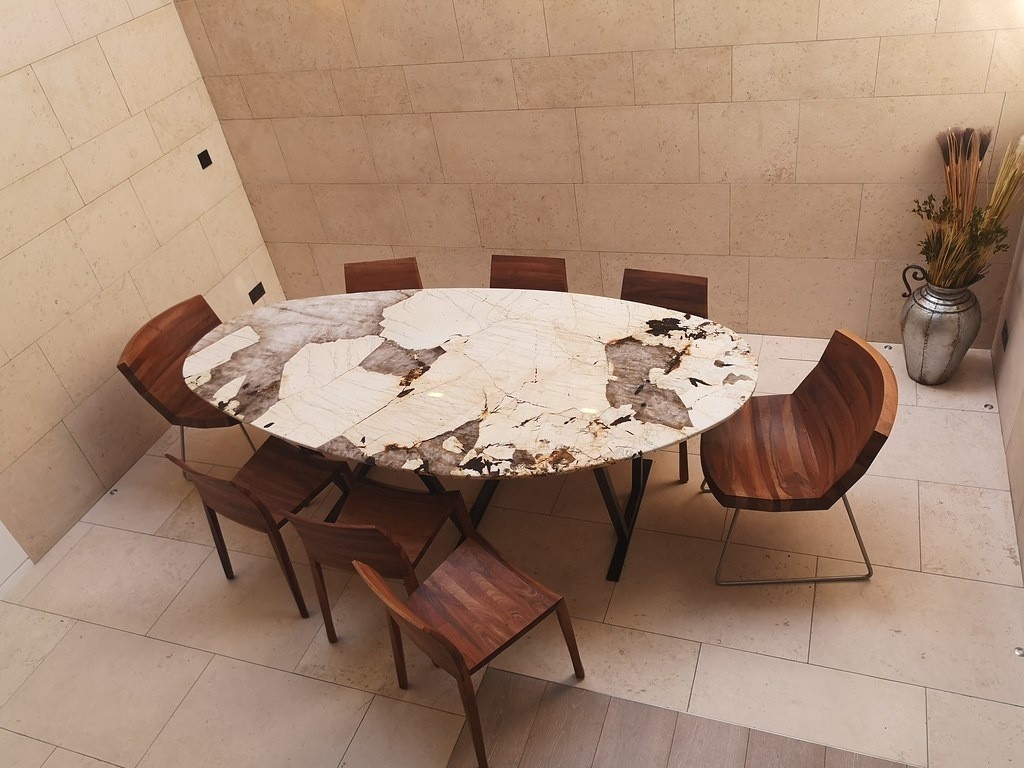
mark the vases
[901,265,982,385]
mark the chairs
[351,532,586,768]
[166,436,351,617]
[699,329,899,586]
[490,255,569,292]
[116,294,256,477]
[344,257,423,293]
[279,476,474,643]
[620,269,708,483]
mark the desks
[183,287,760,581]
